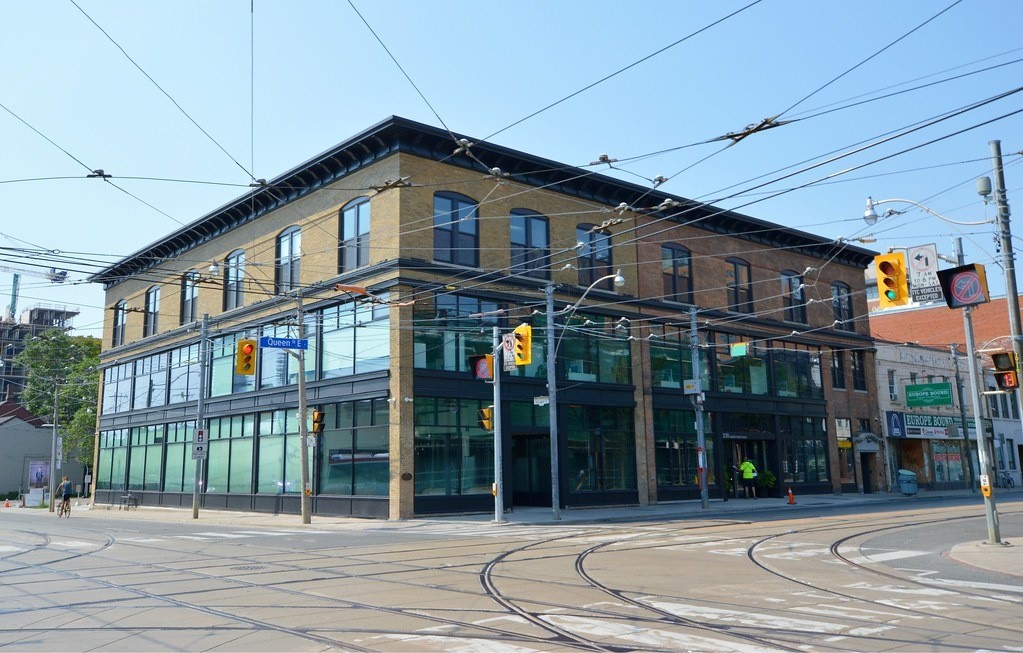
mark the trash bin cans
[899,470,918,495]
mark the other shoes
[754,496,757,500]
[743,495,749,499]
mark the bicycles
[56,493,71,518]
[998,470,1014,488]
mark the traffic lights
[478,409,491,432]
[313,410,325,434]
[237,339,255,375]
[514,325,532,366]
[935,263,991,310]
[990,354,1020,390]
[873,251,909,309]
[468,354,493,381]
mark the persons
[736,457,758,500]
[56,476,74,516]
[35,468,43,488]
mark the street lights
[208,258,311,524]
[862,139,1022,419]
[544,268,627,519]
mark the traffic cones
[5,498,9,507]
[787,486,797,504]
